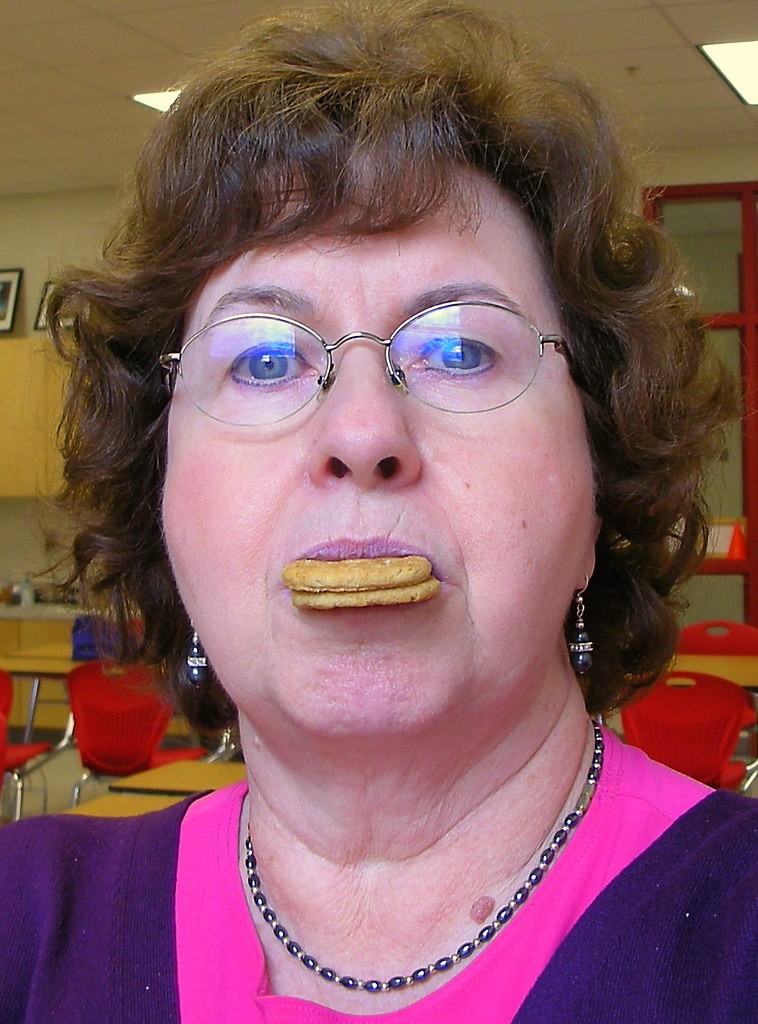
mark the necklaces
[245,718,605,993]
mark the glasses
[159,301,580,426]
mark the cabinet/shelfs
[0,338,89,499]
[0,619,194,738]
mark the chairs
[621,620,758,799]
[0,615,208,821]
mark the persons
[0,2,758,1024]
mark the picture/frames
[34,281,93,330]
[0,268,23,333]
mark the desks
[0,658,185,743]
[64,793,189,817]
[108,760,247,794]
[8,642,71,659]
[622,654,758,695]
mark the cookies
[282,555,432,590]
[291,579,438,609]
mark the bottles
[20,578,34,607]
